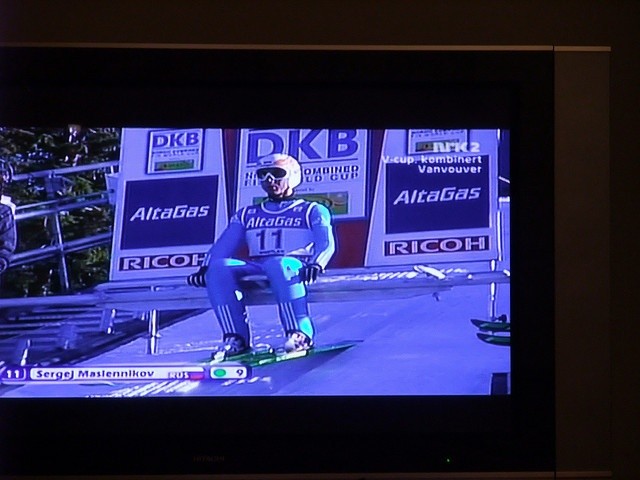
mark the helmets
[256,153,302,188]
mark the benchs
[94,270,510,358]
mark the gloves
[301,264,323,285]
[187,266,208,287]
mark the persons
[0,160,17,277]
[187,154,336,363]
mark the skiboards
[196,339,363,367]
[471,319,511,346]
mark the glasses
[256,167,287,182]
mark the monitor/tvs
[0,40,614,475]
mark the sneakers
[210,336,246,359]
[283,331,310,353]
